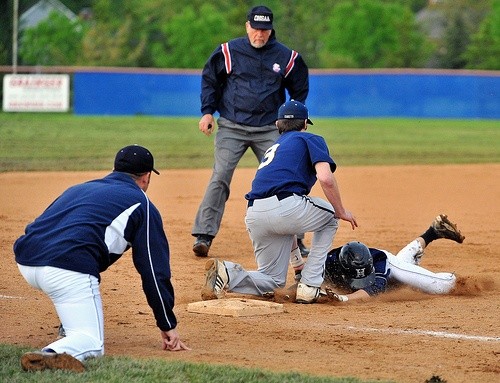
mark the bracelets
[290,248,304,267]
[339,295,348,301]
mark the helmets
[338,241,376,289]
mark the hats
[277,99,313,125]
[248,6,273,29]
[113,145,160,177]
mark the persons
[294,214,465,303]
[192,5,309,256]
[200,103,358,301]
[14,146,193,371]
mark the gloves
[325,285,349,302]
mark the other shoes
[18,351,86,373]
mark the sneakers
[295,283,328,304]
[192,235,212,257]
[293,271,301,280]
[200,258,228,301]
[432,213,466,244]
[297,238,310,257]
